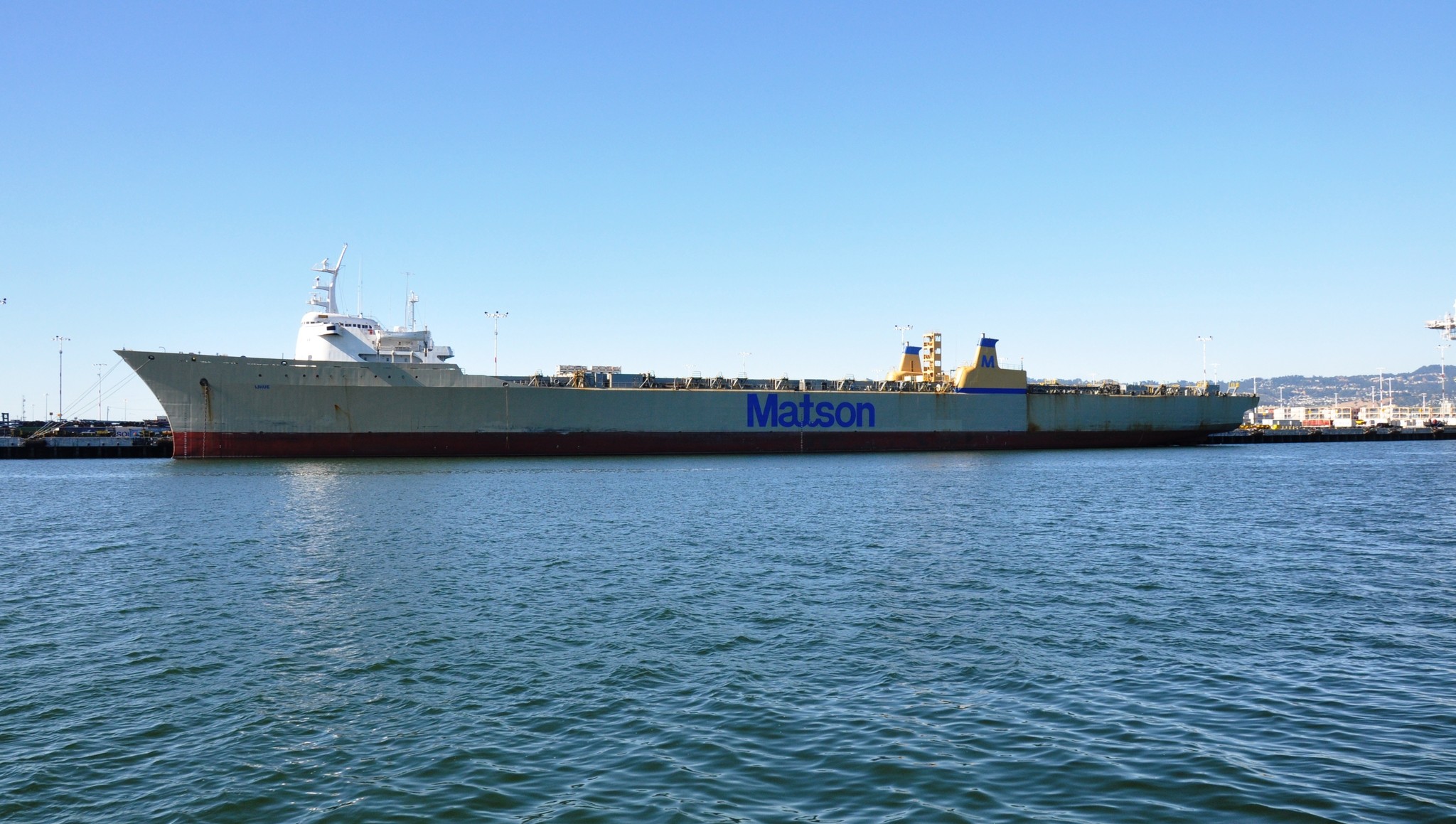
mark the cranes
[1424,299,1456,340]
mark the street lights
[1277,387,1285,408]
[1196,336,1212,387]
[1334,393,1338,407]
[1370,367,1395,424]
[51,335,70,423]
[1435,344,1451,421]
[123,397,128,422]
[94,362,107,423]
[44,393,48,422]
[31,404,34,421]
[1420,393,1427,414]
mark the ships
[114,243,1260,460]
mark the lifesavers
[553,379,559,384]
